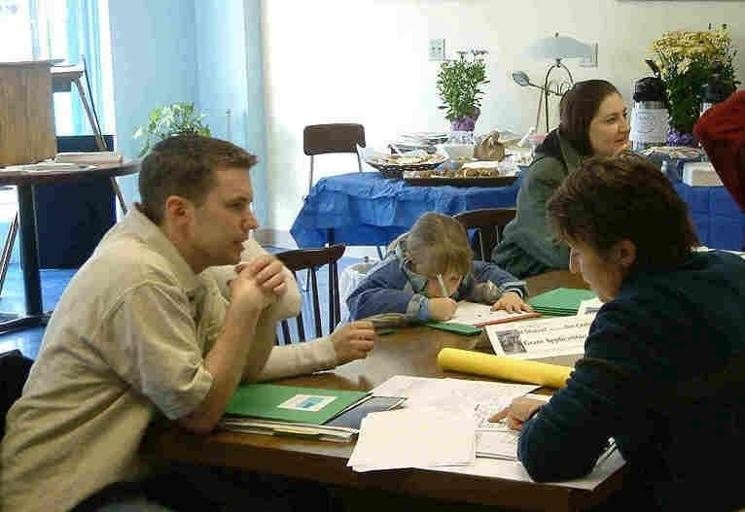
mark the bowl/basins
[437,142,475,159]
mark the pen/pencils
[436,271,449,298]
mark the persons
[1,134,290,511]
[487,154,743,511]
[492,79,634,281]
[344,211,532,324]
[198,233,377,383]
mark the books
[217,383,408,443]
[419,319,485,336]
[525,286,595,316]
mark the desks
[158,268,630,511]
[0,161,145,334]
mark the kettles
[699,60,738,158]
[631,58,670,150]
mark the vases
[666,134,698,149]
[448,112,478,131]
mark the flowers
[651,18,743,133]
[435,47,492,120]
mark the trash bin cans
[19,134,115,269]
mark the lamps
[512,31,595,134]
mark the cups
[661,161,680,185]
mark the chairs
[448,208,519,263]
[269,244,346,348]
[0,348,33,439]
[302,122,384,292]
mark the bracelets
[524,406,539,421]
